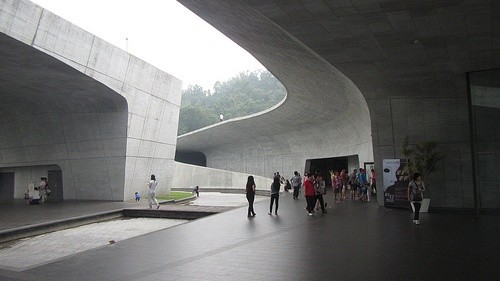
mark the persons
[192,186,200,197]
[147,174,160,209]
[312,173,328,214]
[273,168,378,202]
[135,192,141,202]
[268,176,280,215]
[303,173,315,215]
[408,172,426,224]
[246,176,257,218]
[27,177,50,205]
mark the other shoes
[416,220,420,224]
[413,220,416,222]
